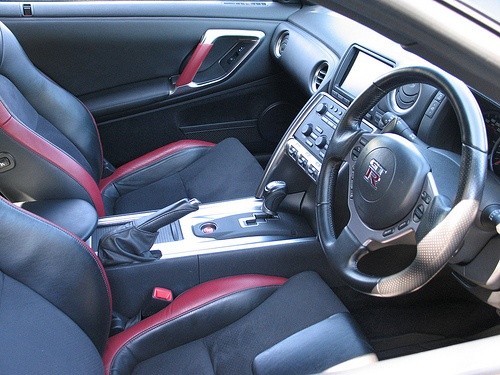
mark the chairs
[0,15,269,223]
[1,196,382,375]
